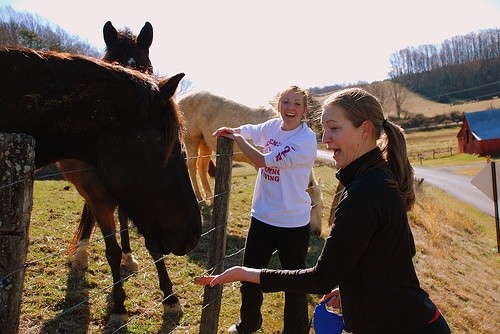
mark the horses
[1,19,326,325]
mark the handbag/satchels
[308,296,344,334]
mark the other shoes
[228,321,260,332]
